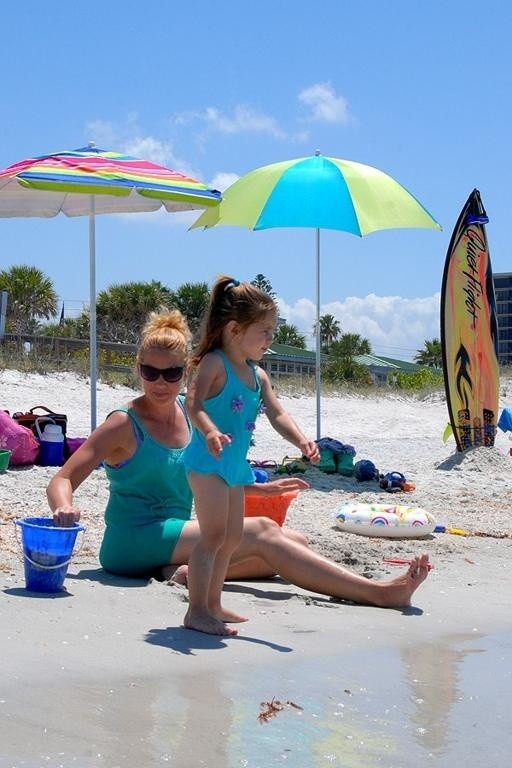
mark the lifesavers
[335,502,435,537]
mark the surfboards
[441,188,501,450]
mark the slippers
[249,459,283,468]
[312,450,355,477]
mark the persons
[178,272,326,641]
[42,302,432,611]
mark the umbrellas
[0,140,222,436]
[186,147,446,442]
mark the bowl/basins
[0,448,12,473]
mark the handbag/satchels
[15,406,67,437]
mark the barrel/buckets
[0,449,11,472]
[35,417,64,466]
[68,438,87,456]
[14,517,86,593]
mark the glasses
[140,364,184,383]
